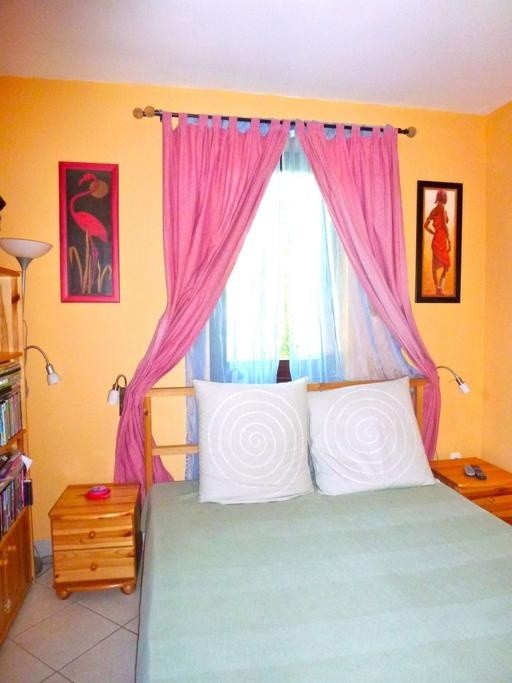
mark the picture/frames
[415,179,465,304]
[57,160,123,307]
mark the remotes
[464,465,475,476]
[471,465,486,479]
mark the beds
[135,372,512,682]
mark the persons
[424,186,454,295]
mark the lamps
[106,374,130,416]
[21,345,61,387]
[1,238,62,575]
[435,365,471,394]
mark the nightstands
[48,480,142,599]
[428,456,512,525]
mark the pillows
[192,375,315,505]
[307,375,440,495]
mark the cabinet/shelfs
[1,265,37,656]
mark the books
[0,389,28,537]
